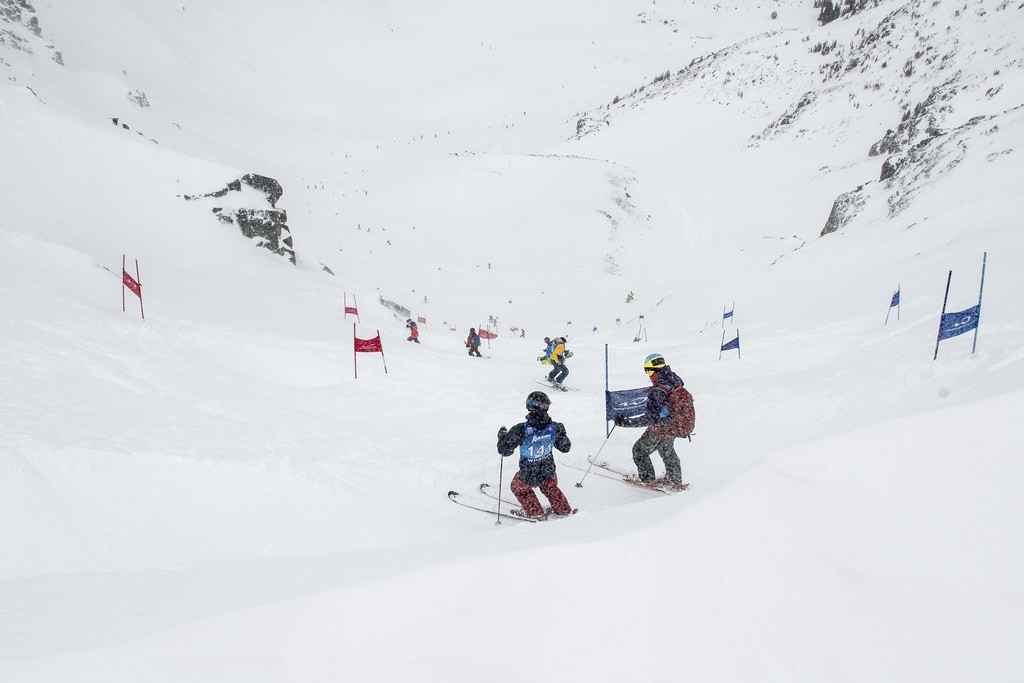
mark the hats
[407,319,412,322]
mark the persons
[466,328,481,357]
[540,337,573,390]
[615,353,695,491]
[497,391,572,519]
[406,318,419,343]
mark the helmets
[644,352,665,371]
[526,391,550,413]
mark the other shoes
[554,381,565,388]
[626,474,652,485]
[511,506,539,517]
[656,478,679,489]
[547,377,553,382]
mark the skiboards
[559,452,689,495]
[447,484,580,524]
[534,375,579,393]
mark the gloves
[406,324,409,327]
[614,413,624,426]
[497,427,508,438]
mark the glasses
[646,369,655,376]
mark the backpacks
[651,378,696,442]
[470,334,480,345]
